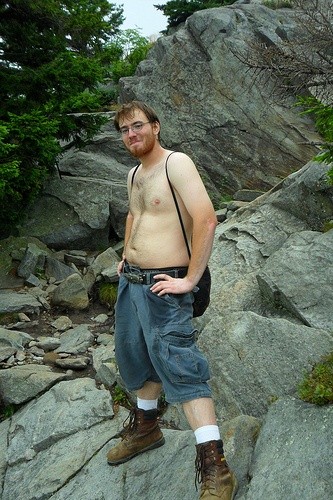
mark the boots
[192,439,239,500]
[105,409,167,465]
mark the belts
[120,259,188,285]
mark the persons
[105,99,239,500]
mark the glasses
[117,122,152,136]
